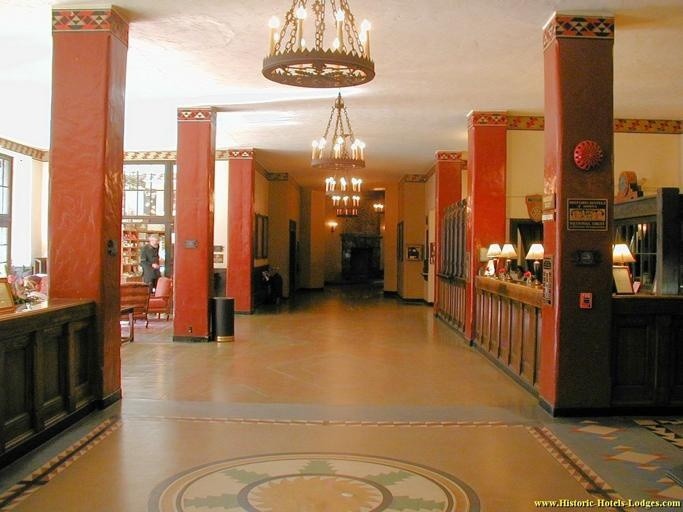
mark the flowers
[2,260,41,303]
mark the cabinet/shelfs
[611,186,682,292]
[122,227,140,281]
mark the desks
[116,301,135,348]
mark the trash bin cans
[212,297,234,342]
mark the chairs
[147,275,175,323]
[119,280,151,329]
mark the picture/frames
[254,213,265,261]
[405,244,424,263]
[261,215,268,261]
[396,223,399,261]
[398,220,403,261]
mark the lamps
[326,222,339,231]
[610,241,636,266]
[373,204,383,214]
[319,171,364,199]
[307,91,368,174]
[499,244,517,280]
[486,241,500,262]
[332,207,359,220]
[525,242,545,286]
[258,0,378,90]
[477,247,493,275]
[325,193,362,210]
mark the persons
[140,233,161,292]
